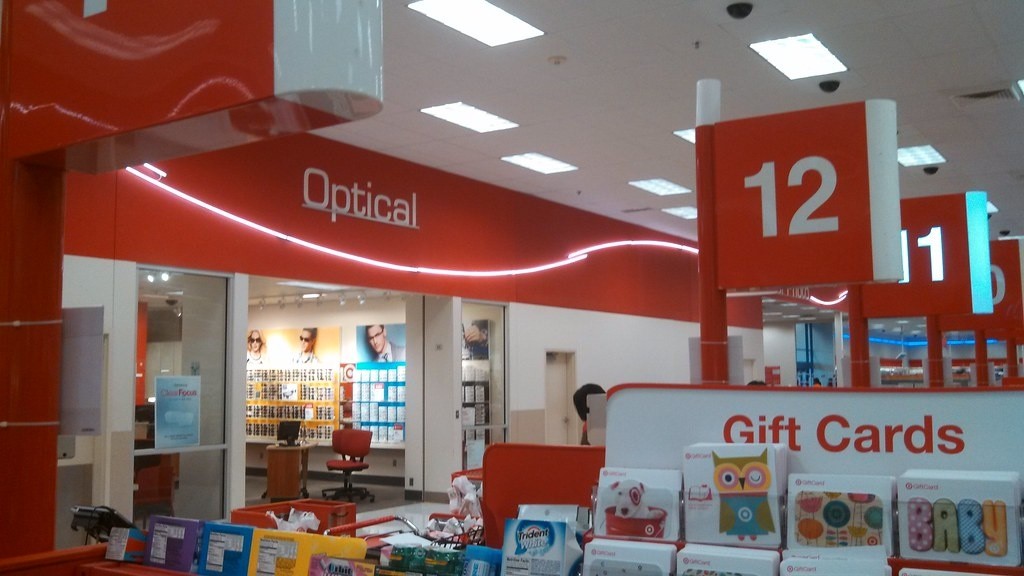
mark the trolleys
[323,514,484,549]
[452,466,483,494]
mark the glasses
[368,331,383,341]
[300,336,309,342]
[251,338,261,343]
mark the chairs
[321,429,376,504]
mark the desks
[267,445,312,501]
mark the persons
[247,329,267,369]
[813,378,821,387]
[365,325,405,361]
[573,384,606,445]
[290,328,321,365]
